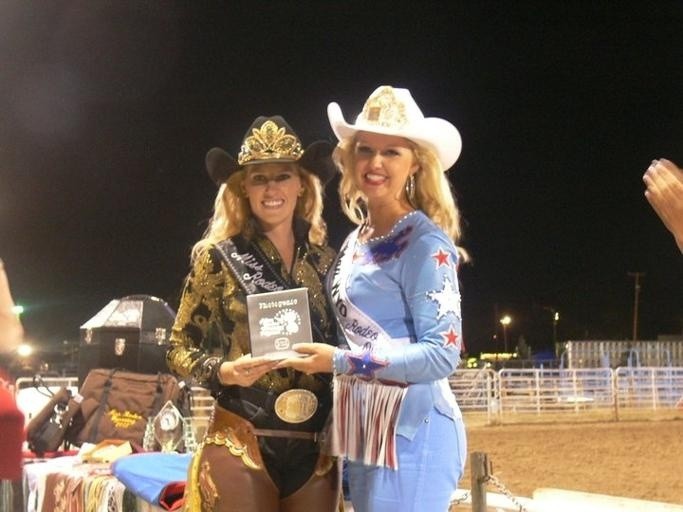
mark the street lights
[550,311,559,363]
[13,303,26,373]
[499,315,511,353]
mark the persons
[317,85,470,510]
[165,115,345,510]
[640,155,683,251]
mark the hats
[325,86,464,175]
[206,115,338,187]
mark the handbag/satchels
[25,387,79,457]
[66,366,180,454]
